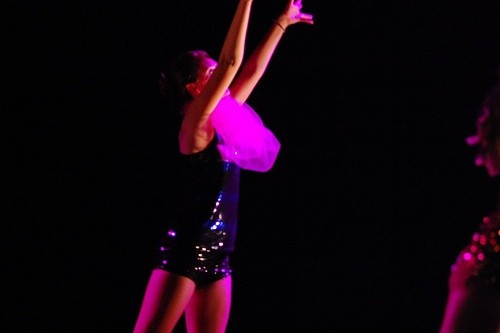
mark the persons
[439,86,500,333]
[132,0,314,333]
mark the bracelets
[274,21,285,32]
